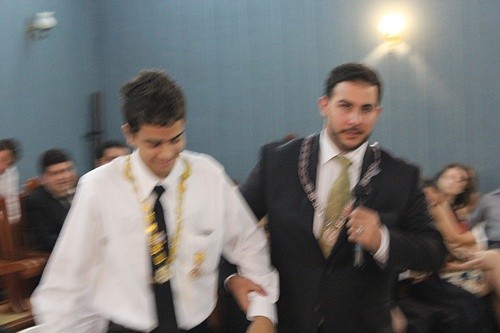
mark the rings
[355,226,364,236]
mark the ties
[315,153,352,262]
[150,186,182,333]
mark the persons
[16,146,83,252]
[29,68,280,333]
[92,141,131,169]
[392,163,500,333]
[0,138,22,238]
[218,63,448,333]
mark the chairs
[0,196,47,312]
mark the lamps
[29,11,57,40]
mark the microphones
[352,184,367,268]
